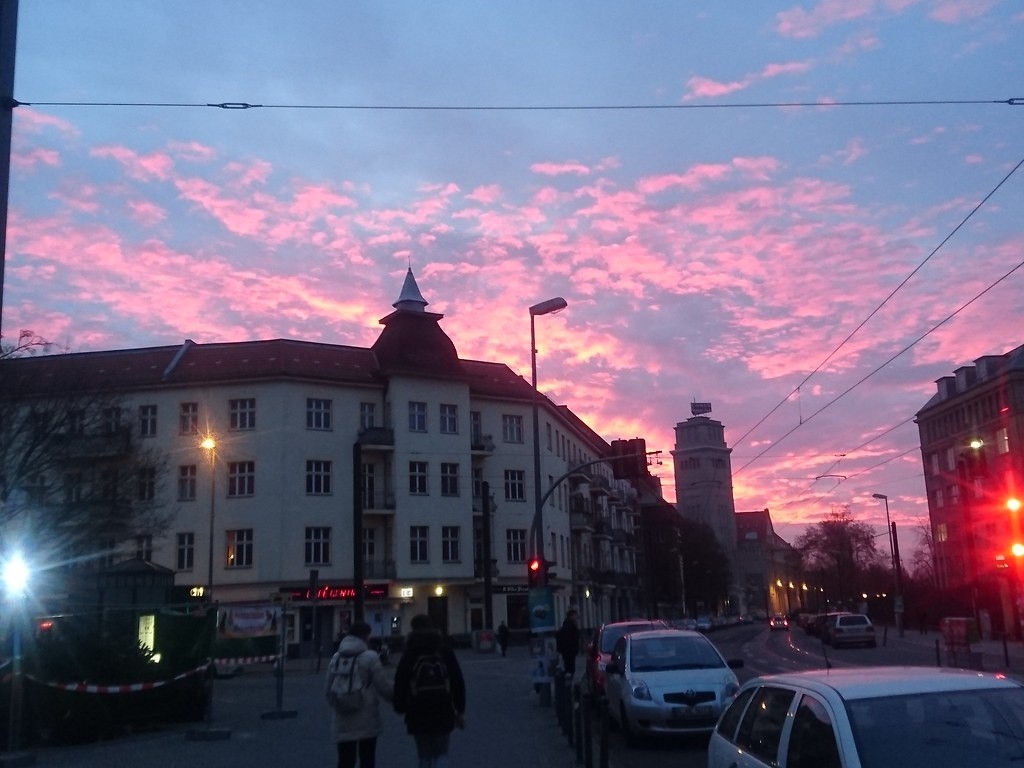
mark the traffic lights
[528,559,539,574]
[540,560,558,587]
[1004,497,1024,519]
[1012,542,1024,557]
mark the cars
[767,615,790,632]
[703,667,1024,768]
[663,610,755,631]
[604,628,746,746]
[586,618,678,698]
[797,611,877,648]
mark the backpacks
[326,649,373,715]
[404,643,453,705]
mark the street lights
[198,435,219,604]
[871,492,903,636]
[0,545,36,768]
[527,295,569,588]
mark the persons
[675,645,703,664]
[557,610,580,672]
[499,621,511,657]
[325,622,394,768]
[392,614,466,768]
[918,608,929,635]
